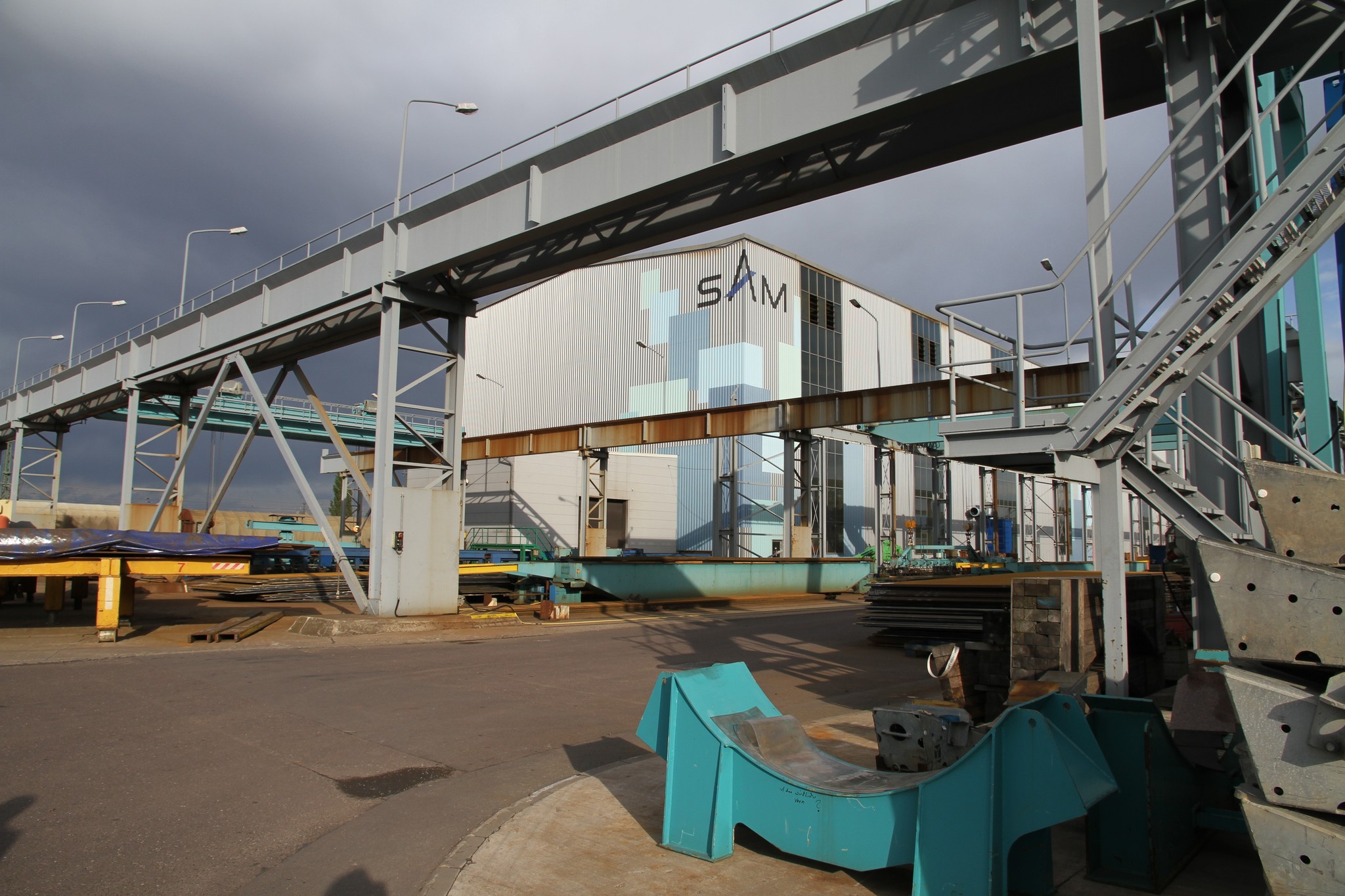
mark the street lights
[1041,257,1072,364]
[475,372,507,435]
[394,98,482,217]
[13,334,66,391]
[69,300,128,369]
[634,339,668,415]
[850,297,882,388]
[178,225,250,317]
